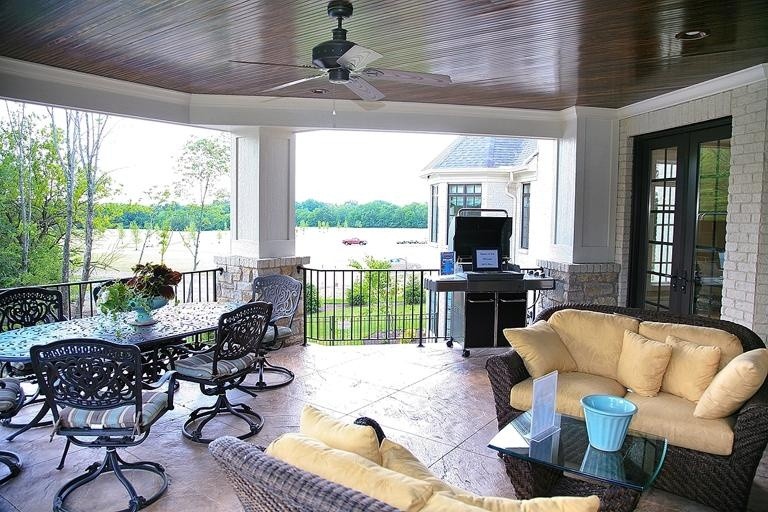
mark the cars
[342,237,367,245]
[396,237,426,245]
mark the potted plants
[93,261,183,341]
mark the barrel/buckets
[578,444,629,484]
[580,392,639,453]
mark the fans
[224,1,453,130]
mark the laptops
[473,248,502,273]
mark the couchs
[203,415,418,512]
[483,302,768,511]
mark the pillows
[502,317,579,382]
[688,347,768,420]
[658,333,724,405]
[615,328,673,399]
[451,487,602,512]
[298,403,384,469]
[378,436,457,497]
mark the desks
[422,269,556,358]
